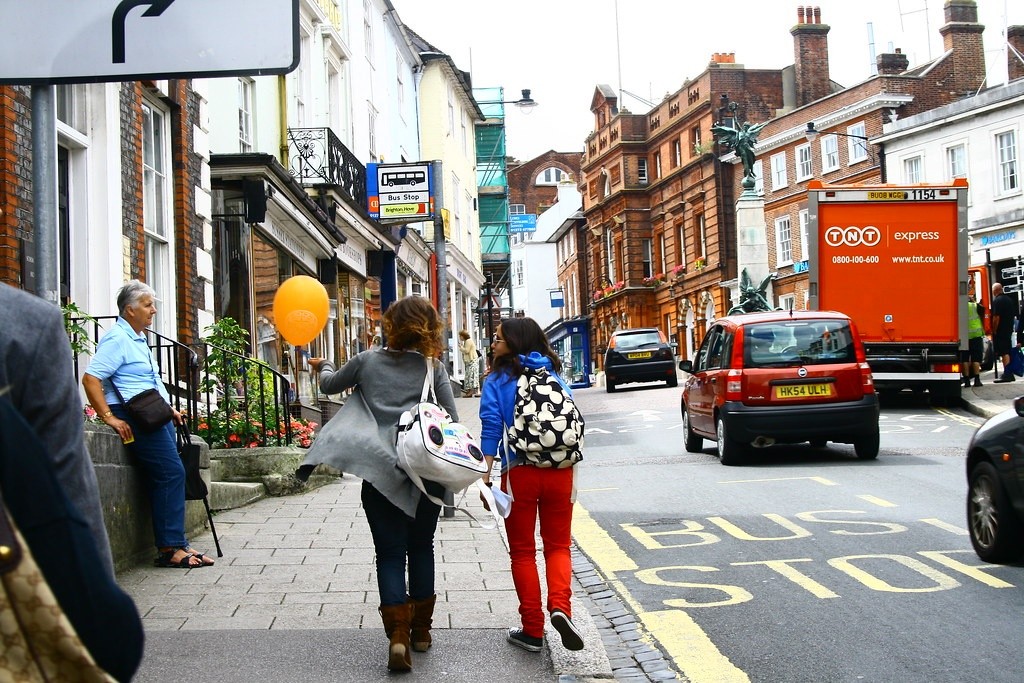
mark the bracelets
[485,482,492,487]
[101,411,113,421]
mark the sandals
[154,547,215,568]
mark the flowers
[695,257,705,270]
[614,281,623,291]
[642,277,654,287]
[605,287,614,296]
[674,264,683,275]
[656,274,665,283]
[594,291,603,300]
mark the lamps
[476,86,541,107]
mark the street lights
[804,123,887,183]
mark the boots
[407,592,436,652]
[378,593,417,670]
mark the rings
[121,428,124,431]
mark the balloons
[273,274,331,348]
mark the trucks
[806,178,992,402]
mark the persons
[305,296,460,672]
[455,330,482,397]
[0,281,144,683]
[480,317,586,652]
[733,111,758,179]
[82,280,214,567]
[962,283,1024,387]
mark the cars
[966,396,1024,560]
[678,310,880,465]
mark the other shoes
[974,378,983,387]
[463,392,472,398]
[993,374,1015,382]
[964,381,972,388]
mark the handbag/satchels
[122,387,173,433]
[173,420,208,501]
[395,356,489,494]
[1009,345,1024,376]
[476,350,482,357]
[0,524,121,683]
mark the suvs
[600,327,678,393]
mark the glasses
[492,332,507,345]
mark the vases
[644,283,652,287]
[661,277,666,283]
[677,269,684,275]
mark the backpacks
[504,364,585,468]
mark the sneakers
[506,626,544,652]
[551,608,585,651]
